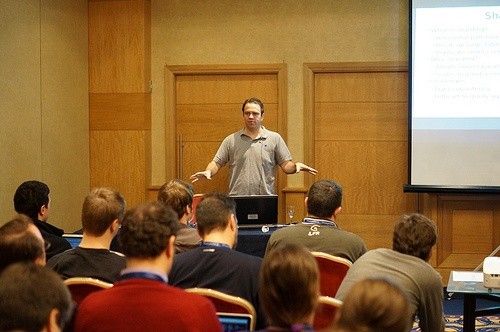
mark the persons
[190,97,318,195]
[0,179,444,332]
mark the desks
[447,270,500,332]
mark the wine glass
[287,205,296,226]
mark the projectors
[483,257,500,289]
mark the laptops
[230,195,278,228]
[62,234,83,249]
[217,312,253,332]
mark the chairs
[58,250,353,332]
